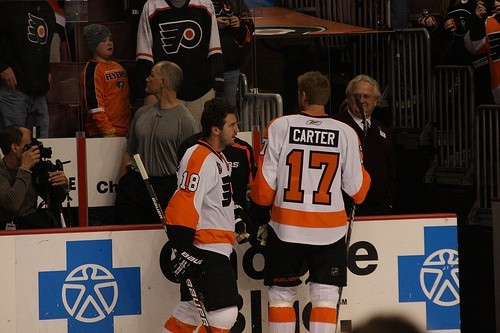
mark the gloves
[213,73,225,93]
[170,247,205,282]
[137,58,153,83]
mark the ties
[360,121,369,131]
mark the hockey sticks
[133,154,213,333]
[336,94,368,333]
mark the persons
[335,75,399,216]
[164,98,239,333]
[212,0,255,125]
[113,61,198,226]
[417,0,500,114]
[177,97,256,225]
[0,125,70,230]
[132,0,224,131]
[249,72,371,333]
[0,0,56,137]
[80,24,132,137]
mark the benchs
[43,0,146,138]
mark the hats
[82,24,112,49]
